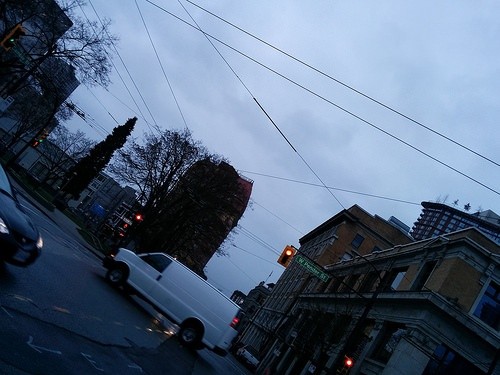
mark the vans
[102,246,244,357]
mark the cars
[0,162,43,268]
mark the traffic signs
[295,255,329,283]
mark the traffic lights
[277,244,297,269]
[32,131,50,148]
